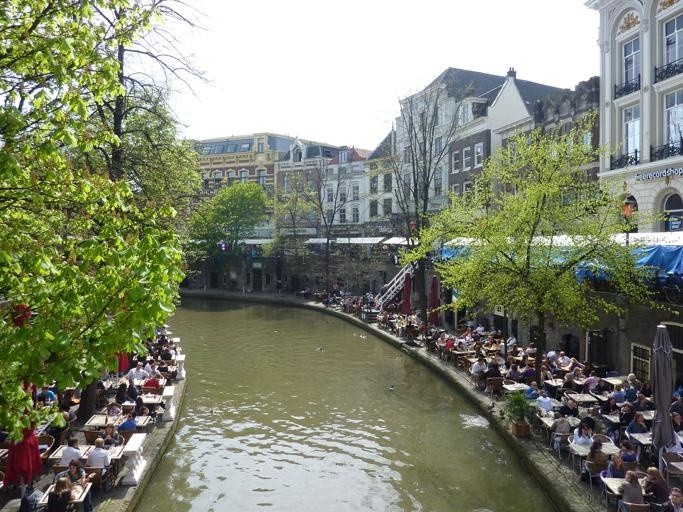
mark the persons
[300,286,421,341]
[19,326,177,511]
[420,318,585,382]
[617,467,683,512]
[521,368,683,481]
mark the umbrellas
[399,274,414,329]
[429,274,442,330]
[2,415,44,499]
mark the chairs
[380,312,504,397]
[0,359,177,511]
[504,344,682,511]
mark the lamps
[663,175,670,187]
